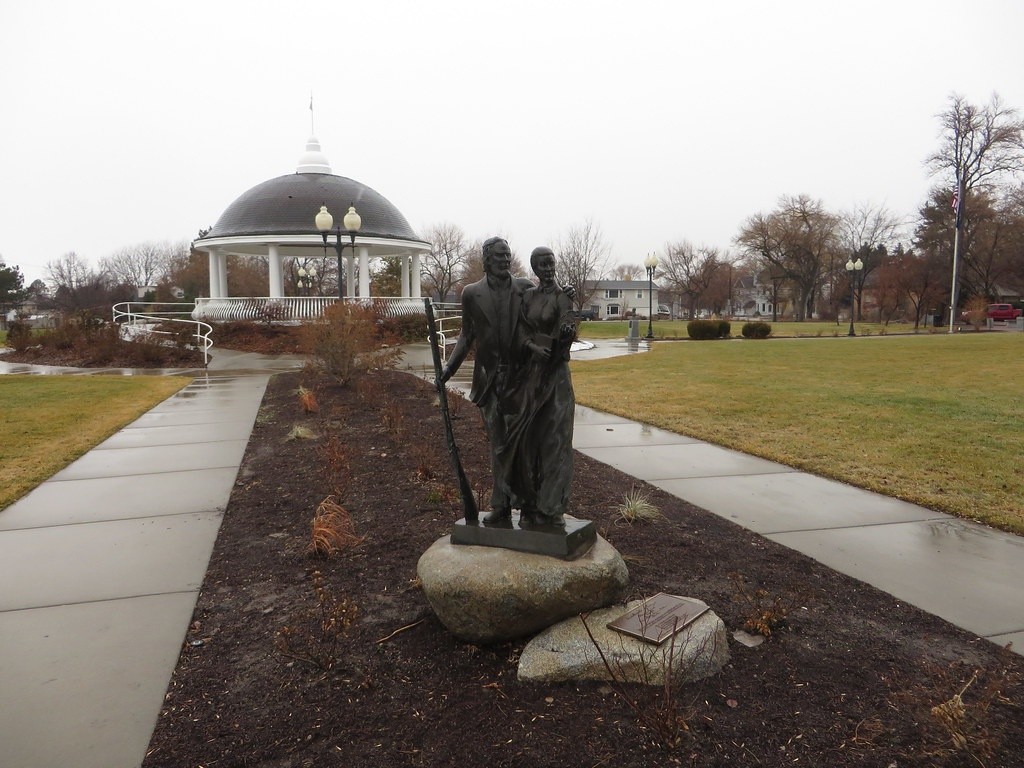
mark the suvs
[573,309,594,322]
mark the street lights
[643,250,659,339]
[297,265,317,297]
[315,202,363,303]
[845,257,864,336]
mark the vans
[658,304,671,319]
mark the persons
[434,237,577,527]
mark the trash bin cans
[933,315,944,327]
[628,320,639,338]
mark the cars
[960,303,1024,326]
[677,311,705,320]
